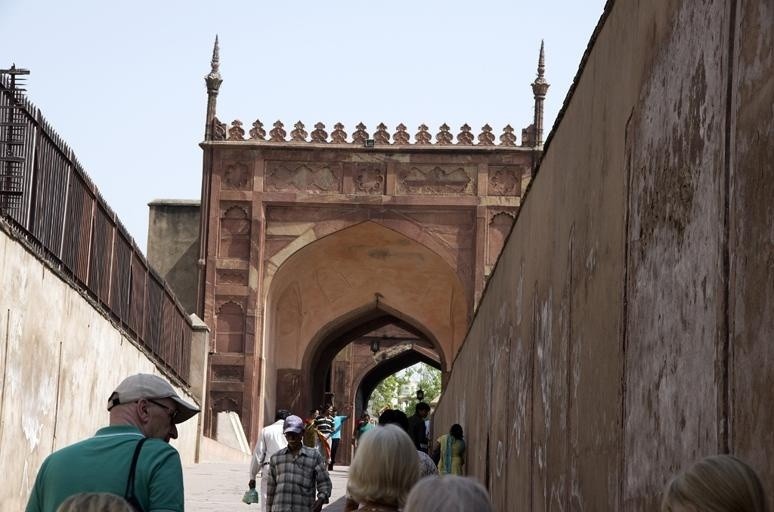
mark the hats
[103,370,204,424]
[275,408,290,419]
[282,414,305,436]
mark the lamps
[370,342,379,355]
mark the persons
[248,401,494,512]
[22,373,201,512]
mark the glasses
[144,395,181,420]
